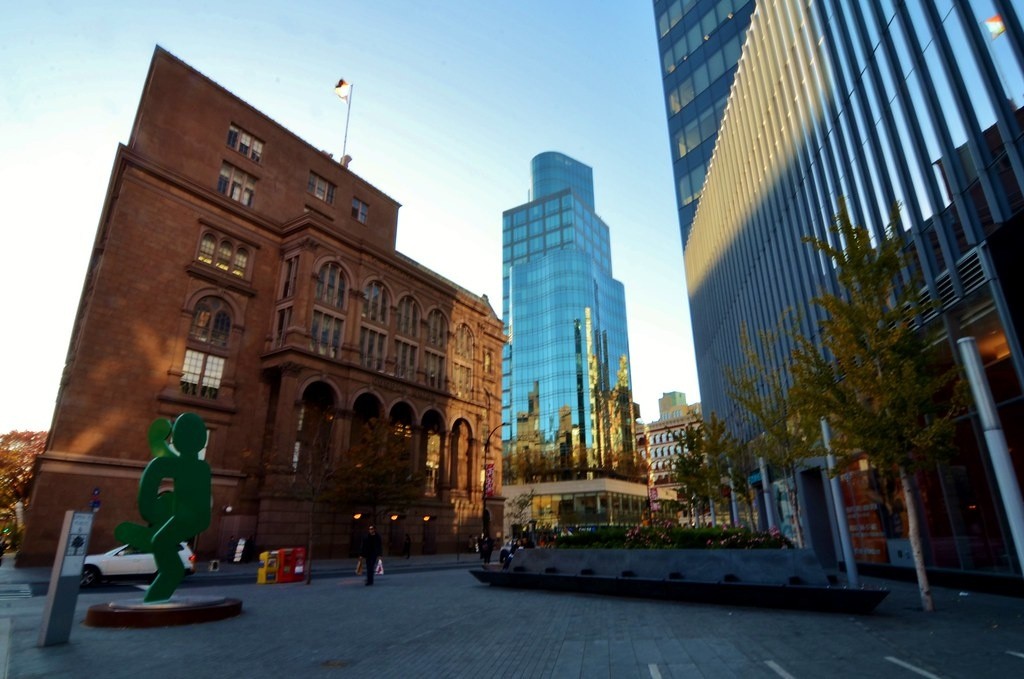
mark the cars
[79,540,198,590]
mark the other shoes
[481,565,487,569]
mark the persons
[359,526,382,586]
[468,533,534,571]
[401,533,411,559]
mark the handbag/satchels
[374,559,384,576]
[355,559,363,576]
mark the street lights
[482,420,512,539]
[646,455,667,526]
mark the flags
[334,78,352,104]
[985,12,1006,39]
